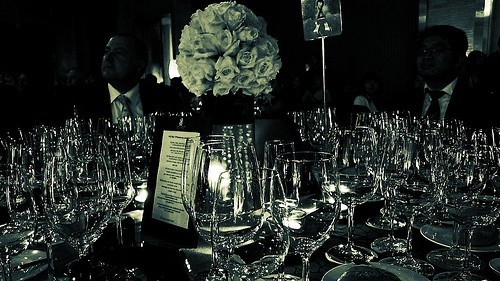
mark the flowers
[177,0,283,98]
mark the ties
[117,95,132,117]
[424,89,447,119]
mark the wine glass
[0,102,500,281]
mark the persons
[78,31,168,121]
[313,0,331,33]
[406,23,490,127]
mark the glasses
[424,47,445,55]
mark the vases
[203,92,256,207]
